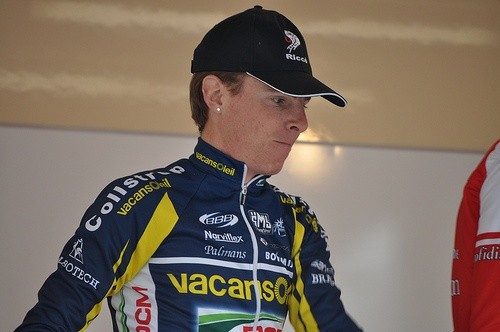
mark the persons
[451,136,499,332]
[12,5,362,332]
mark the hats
[191,2,347,108]
